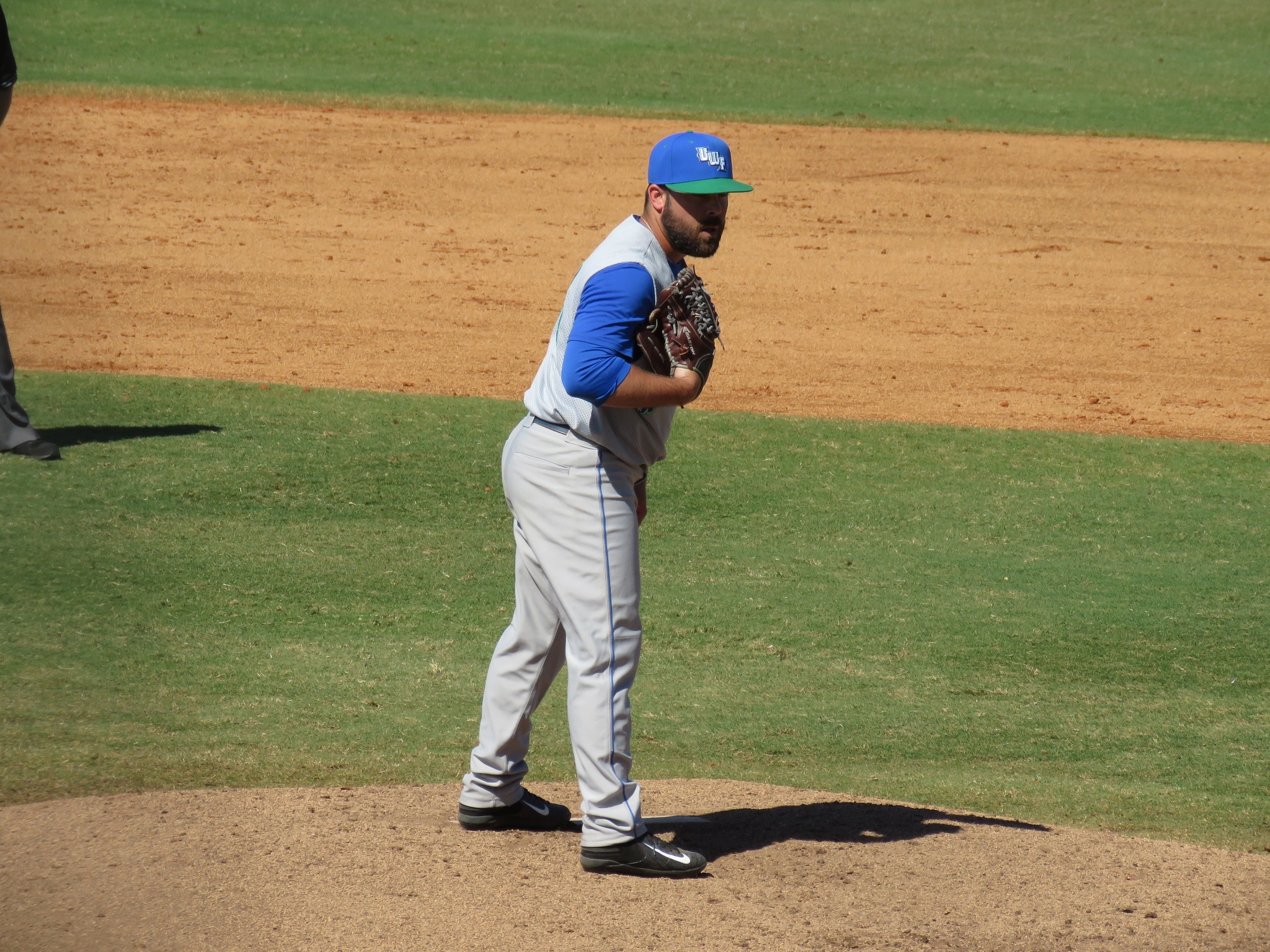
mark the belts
[533,417,570,435]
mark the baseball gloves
[637,268,721,400]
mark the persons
[0,5,61,462]
[456,131,753,877]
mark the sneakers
[459,788,570,831]
[580,832,706,876]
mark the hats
[646,131,754,194]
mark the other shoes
[7,439,61,461]
